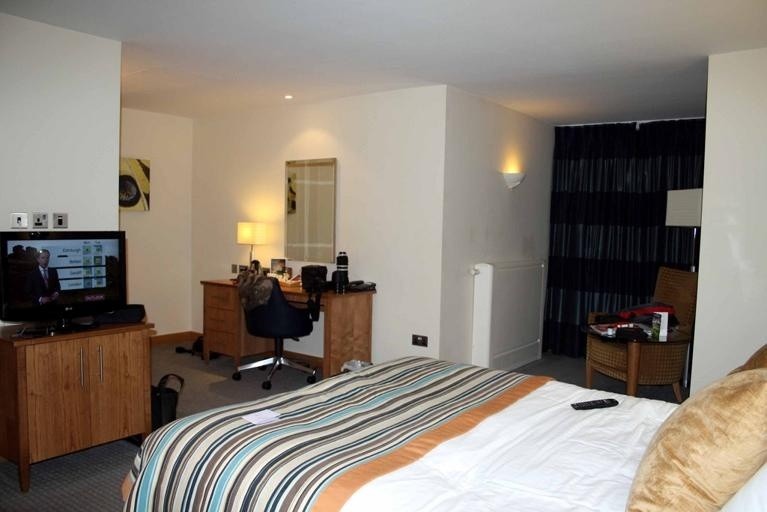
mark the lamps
[236,222,268,269]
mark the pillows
[626,341,767,512]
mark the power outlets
[32,211,48,228]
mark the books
[651,312,668,338]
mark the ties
[43,269,49,285]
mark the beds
[120,355,767,512]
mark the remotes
[572,397,619,411]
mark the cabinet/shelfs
[3,321,155,464]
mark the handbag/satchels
[134,374,184,445]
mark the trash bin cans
[340,359,373,375]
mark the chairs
[231,278,319,390]
[586,266,699,403]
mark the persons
[8,245,61,304]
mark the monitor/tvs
[0,230,127,325]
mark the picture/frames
[120,156,150,211]
[285,158,336,264]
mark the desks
[201,278,376,381]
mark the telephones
[348,280,377,293]
